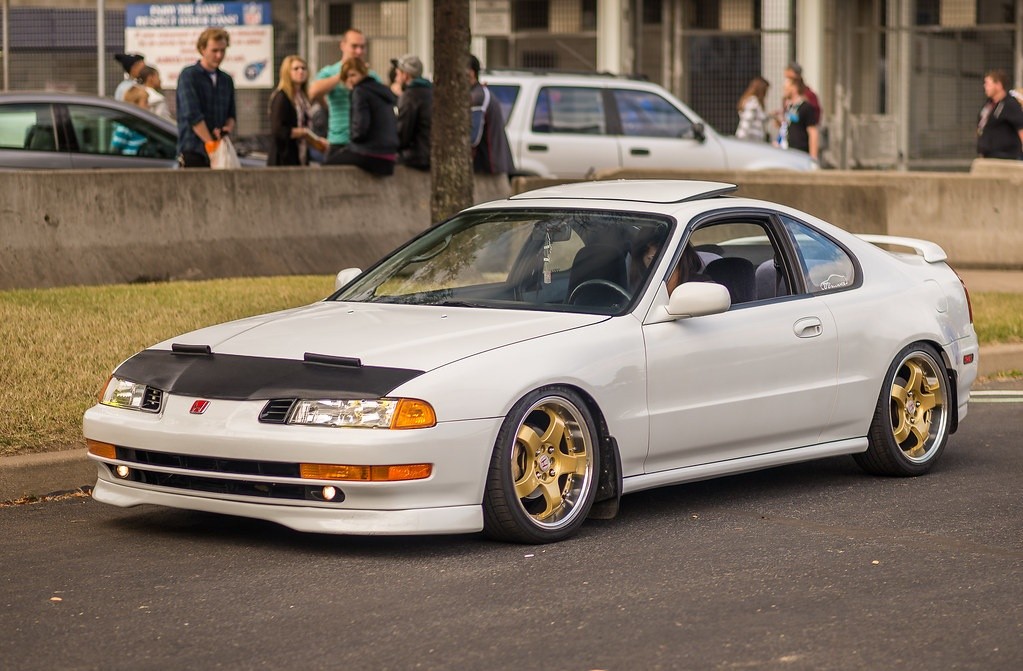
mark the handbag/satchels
[205,133,241,170]
[469,84,492,147]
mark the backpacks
[124,84,148,110]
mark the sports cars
[80,178,979,549]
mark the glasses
[291,66,306,70]
[645,254,655,268]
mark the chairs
[564,244,849,307]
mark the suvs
[475,65,822,180]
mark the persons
[174,25,237,167]
[734,77,773,141]
[110,86,150,155]
[628,224,711,298]
[469,54,516,174]
[325,56,399,177]
[775,62,821,158]
[266,55,330,166]
[309,29,384,150]
[113,52,171,117]
[393,54,433,169]
[976,69,1023,160]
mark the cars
[0,88,266,168]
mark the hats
[115,53,144,71]
[398,54,423,77]
